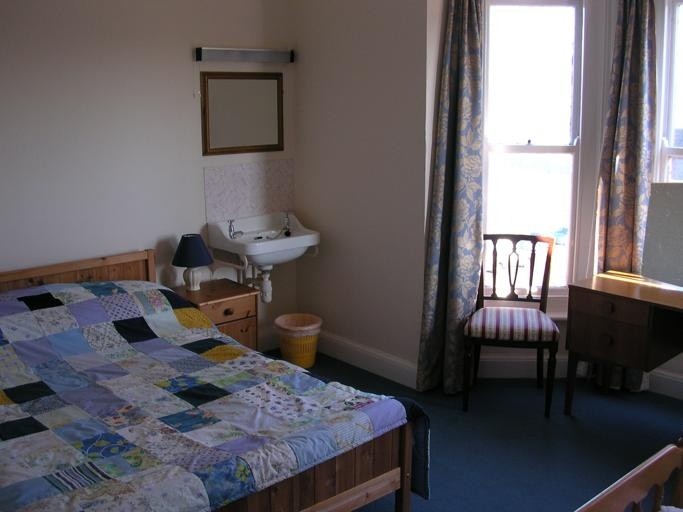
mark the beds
[0,249,415,511]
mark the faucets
[228,219,244,239]
[282,212,292,231]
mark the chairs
[461,233,561,420]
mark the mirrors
[198,71,284,157]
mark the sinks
[208,211,321,266]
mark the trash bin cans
[274,312,323,369]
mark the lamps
[171,233,214,292]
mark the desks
[563,269,682,418]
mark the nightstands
[168,277,261,352]
